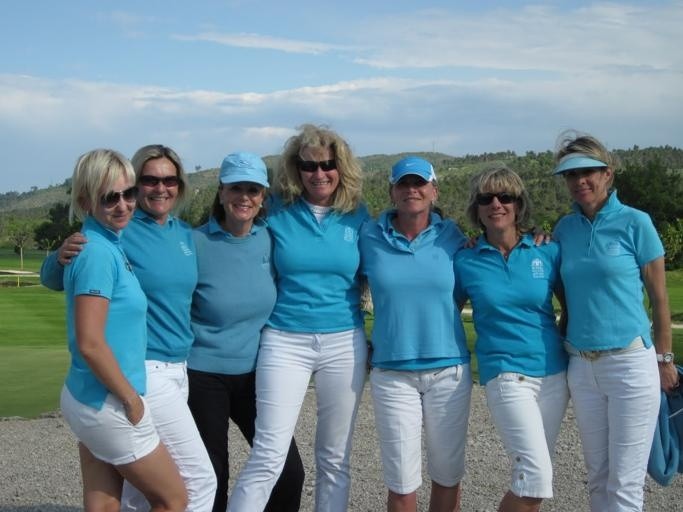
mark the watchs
[655,351,676,364]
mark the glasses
[142,174,180,186]
[100,186,138,206]
[476,192,514,204]
[298,159,337,172]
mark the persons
[224,121,371,512]
[39,143,219,512]
[462,135,679,512]
[60,148,190,512]
[190,151,307,512]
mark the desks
[0,272,41,287]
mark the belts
[563,336,645,358]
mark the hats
[389,157,436,184]
[551,153,607,176]
[219,151,270,189]
